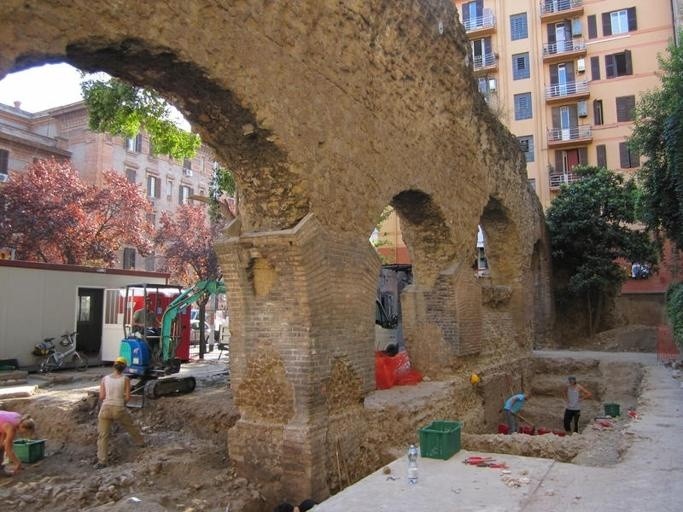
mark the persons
[504,392,531,433]
[130,298,162,336]
[561,374,592,433]
[92,357,147,471]
[0,410,36,472]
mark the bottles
[407,444,418,484]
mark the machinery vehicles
[110,277,235,399]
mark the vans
[371,293,399,355]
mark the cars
[189,310,229,348]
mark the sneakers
[95,463,109,469]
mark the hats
[116,357,128,367]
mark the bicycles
[30,328,95,373]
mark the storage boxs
[603,404,620,418]
[12,439,48,463]
[417,420,461,460]
